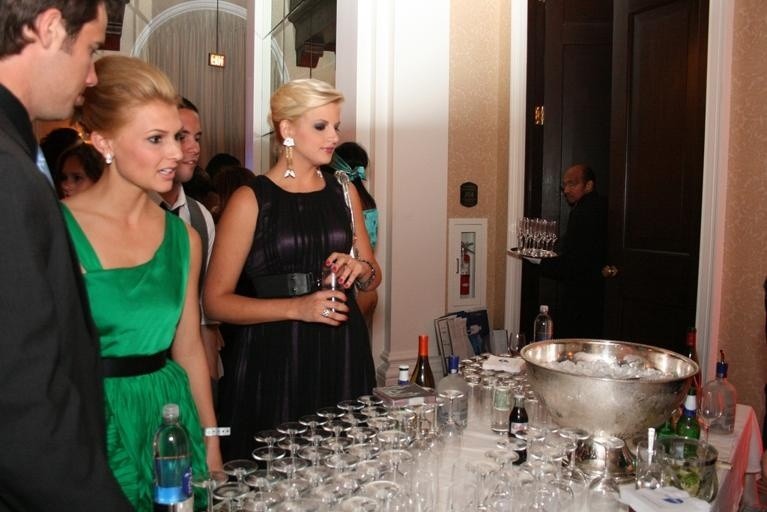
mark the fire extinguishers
[460,240,475,295]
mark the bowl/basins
[520,338,700,441]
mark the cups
[657,435,704,492]
[698,445,719,502]
[319,259,344,317]
[633,441,663,491]
[459,352,560,431]
[508,331,525,355]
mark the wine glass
[191,387,461,512]
[447,424,626,511]
[515,218,556,257]
[696,385,721,449]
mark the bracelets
[352,259,376,291]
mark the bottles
[436,355,468,430]
[396,364,410,387]
[694,361,734,431]
[683,327,700,390]
[675,389,700,457]
[151,402,191,512]
[655,413,673,454]
[411,334,435,390]
[533,305,552,341]
[508,395,527,464]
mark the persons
[41,53,256,512]
[524,162,606,341]
[330,141,378,317]
[201,77,383,495]
[0,0,133,511]
